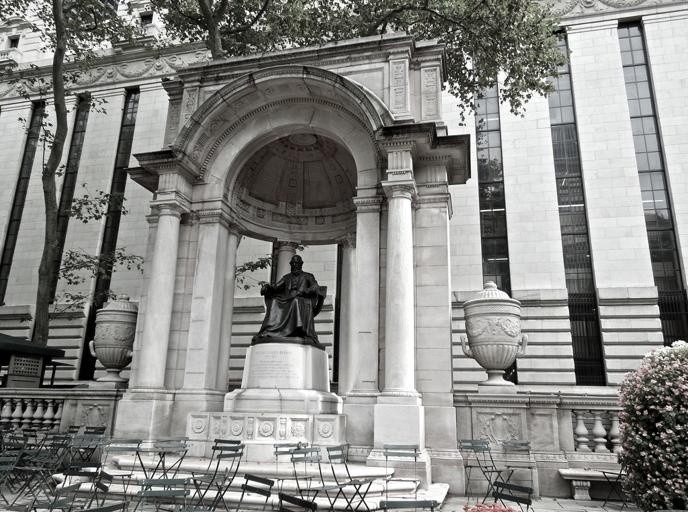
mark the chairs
[0,421,533,512]
[598,461,636,511]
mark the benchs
[557,468,631,500]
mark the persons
[254,254,321,339]
